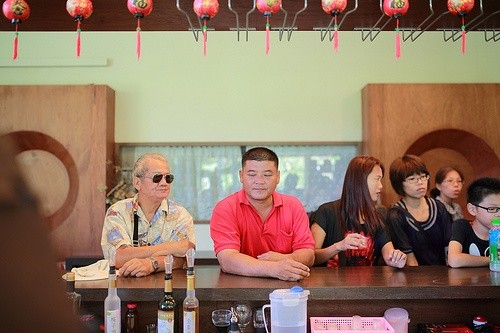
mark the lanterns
[256,0,281,54]
[66,0,93,56]
[2,0,30,60]
[127,0,154,60]
[447,0,474,53]
[383,0,409,59]
[321,0,347,52]
[193,0,220,56]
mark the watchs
[147,256,159,273]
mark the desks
[69,262,500,333]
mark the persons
[387,154,454,266]
[447,177,500,267]
[100,153,197,278]
[309,155,407,268]
[210,147,315,282]
[430,165,464,220]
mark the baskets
[310,315,395,333]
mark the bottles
[126,303,137,333]
[104,265,122,333]
[55,260,104,333]
[229,306,238,331]
[384,308,409,333]
[489,215,500,272]
[182,248,199,333]
[473,317,490,333]
[156,254,178,333]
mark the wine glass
[235,304,252,333]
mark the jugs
[262,286,310,333]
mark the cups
[253,306,269,333]
[211,310,231,333]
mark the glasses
[403,174,430,184]
[472,204,499,213]
[143,173,174,184]
